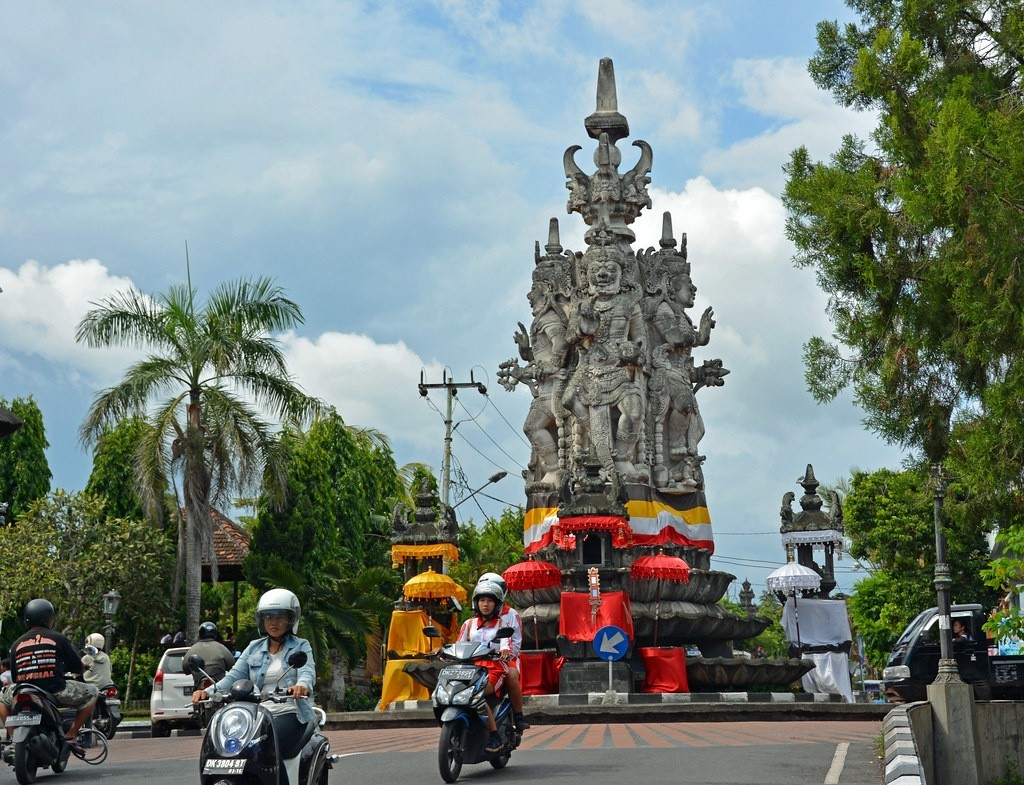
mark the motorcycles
[181,650,340,785]
[92,684,127,738]
[2,682,69,785]
[421,622,525,783]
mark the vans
[149,646,238,739]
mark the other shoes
[71,743,85,755]
[485,738,504,752]
[514,716,530,731]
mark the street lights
[918,403,969,684]
[101,589,121,660]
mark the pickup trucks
[881,603,1024,703]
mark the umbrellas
[403,565,468,617]
[858,634,864,666]
[631,549,690,648]
[502,555,562,650]
[767,556,822,653]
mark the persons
[478,572,530,729]
[0,598,233,756]
[191,588,316,785]
[754,645,767,659]
[513,210,716,493]
[432,581,514,752]
[950,620,973,643]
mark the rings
[194,694,196,695]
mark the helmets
[256,589,301,637]
[85,633,105,650]
[472,573,506,613]
[25,599,55,627]
[199,622,217,639]
